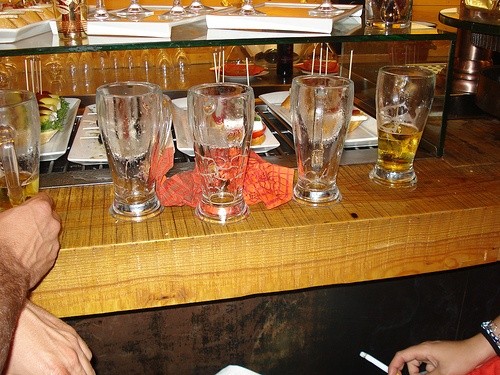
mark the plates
[67,103,150,166]
[209,66,270,81]
[16,98,81,160]
[297,68,339,76]
[258,91,379,148]
[171,97,280,157]
[0,20,51,44]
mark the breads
[0,6,62,29]
[281,95,364,133]
[11,129,59,146]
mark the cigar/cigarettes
[360,352,400,375]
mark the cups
[187,82,254,224]
[291,76,355,207]
[0,89,41,211]
[53,0,88,39]
[367,66,436,188]
[96,81,171,222]
[364,0,413,29]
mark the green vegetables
[40,97,71,132]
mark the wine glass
[307,0,345,16]
[87,0,267,18]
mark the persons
[0,192,96,375]
[390,315,500,375]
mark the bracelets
[480,319,500,357]
